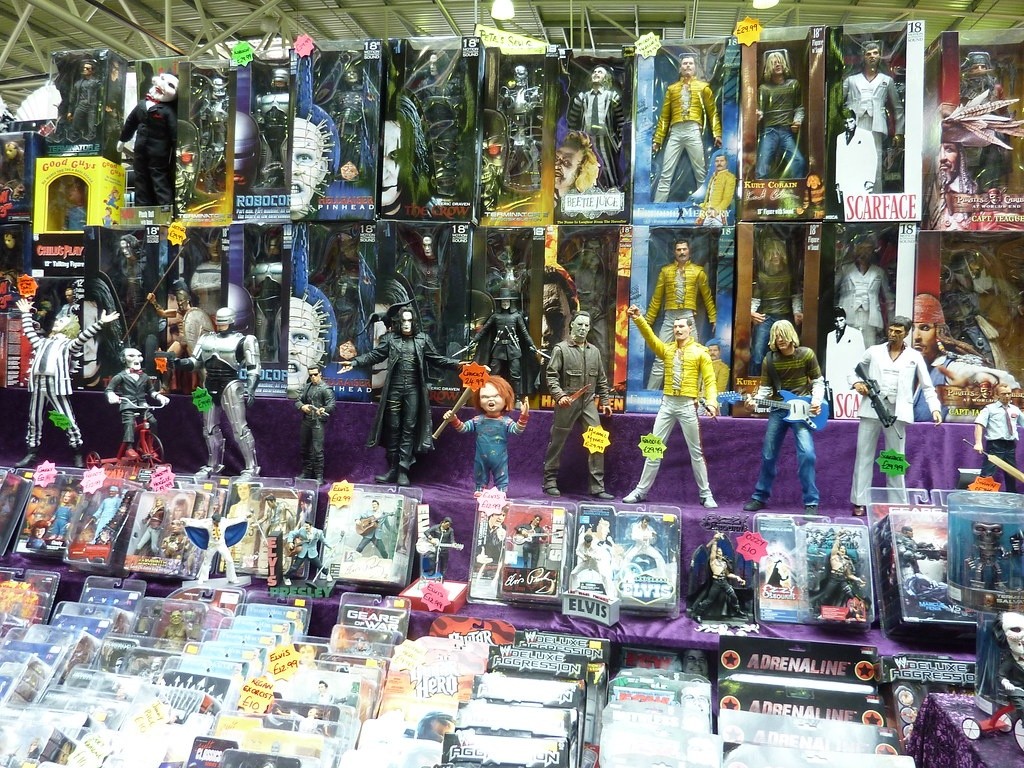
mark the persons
[913,294,1022,423]
[476,505,509,564]
[543,310,615,499]
[294,365,336,486]
[744,320,825,521]
[105,349,170,462]
[924,104,1024,231]
[175,308,261,484]
[554,131,599,198]
[622,305,718,508]
[0,39,1024,768]
[443,375,529,498]
[973,386,1024,493]
[826,307,865,390]
[835,111,877,195]
[14,298,120,469]
[337,306,472,486]
[468,288,539,410]
[846,316,943,516]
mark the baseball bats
[431,365,491,440]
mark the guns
[904,545,947,561]
[154,350,177,367]
[308,405,329,417]
[854,362,903,439]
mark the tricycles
[961,687,1024,751]
[85,397,165,472]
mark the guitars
[513,529,559,545]
[284,538,311,557]
[715,388,829,431]
[355,513,395,535]
[416,537,465,555]
